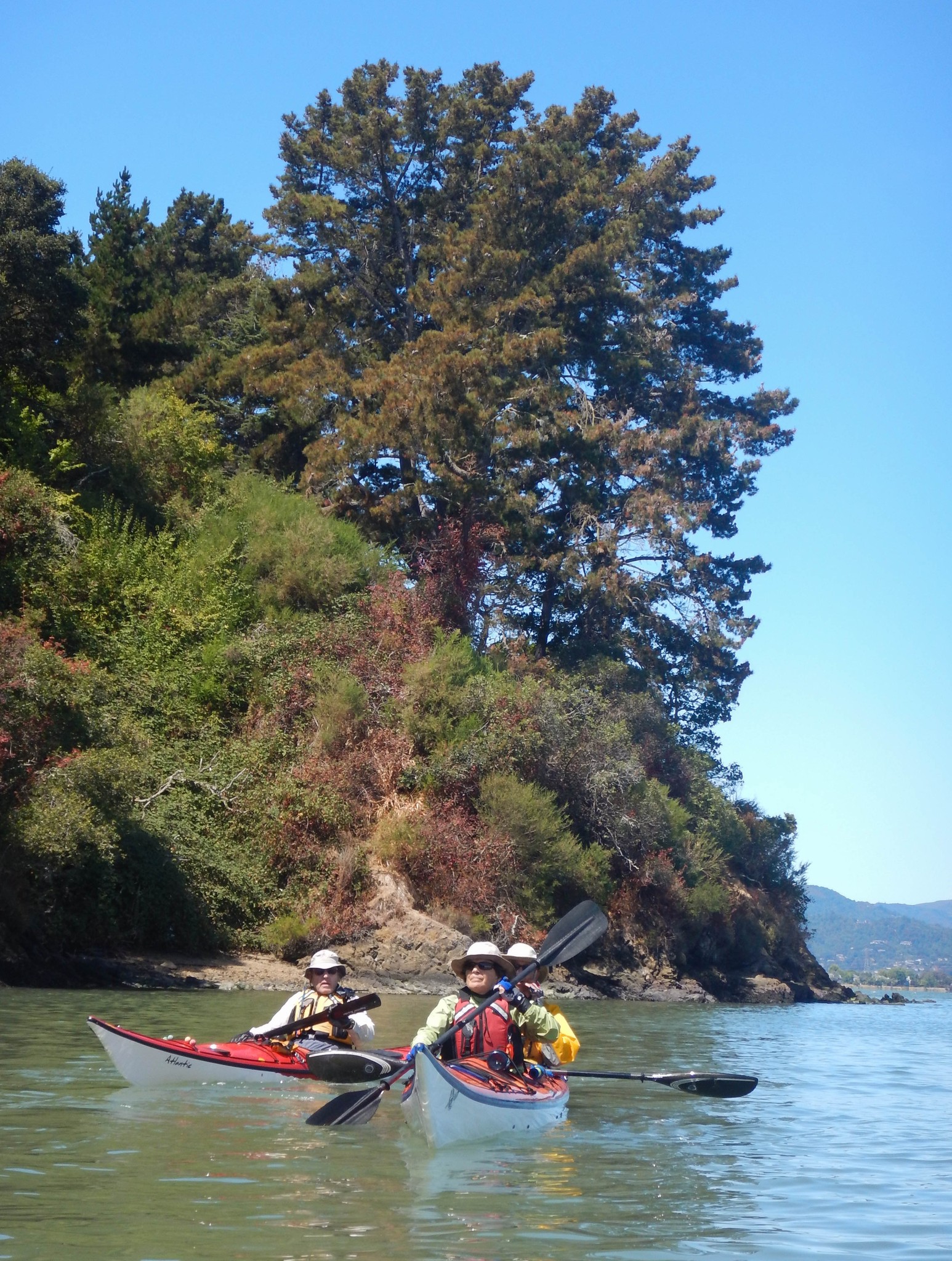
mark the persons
[406,941,581,1067]
[229,949,376,1051]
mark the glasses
[464,961,496,971]
[512,964,529,971]
[312,968,337,975]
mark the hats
[451,942,514,982]
[301,950,346,978]
[500,942,549,984]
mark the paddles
[245,992,382,1040]
[305,899,609,1126]
[305,1051,759,1099]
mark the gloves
[231,1031,253,1043]
[326,1003,351,1029]
[406,1042,427,1061]
[492,977,526,1008]
[523,981,544,1007]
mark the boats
[399,1040,572,1146]
[82,1015,419,1113]
